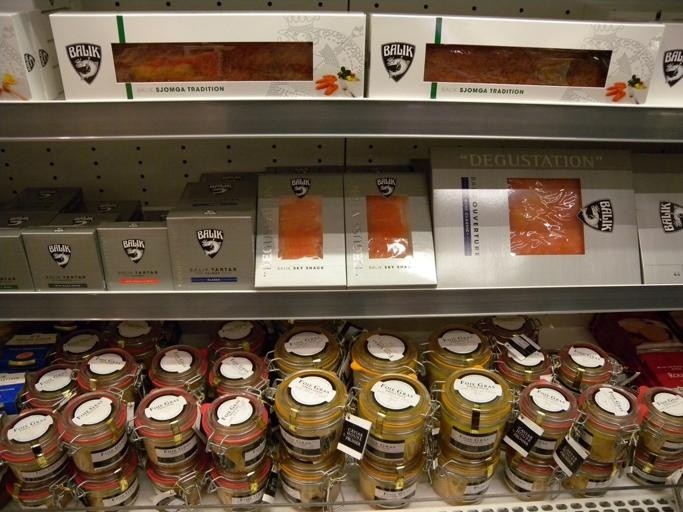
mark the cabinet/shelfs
[0,1,683,512]
